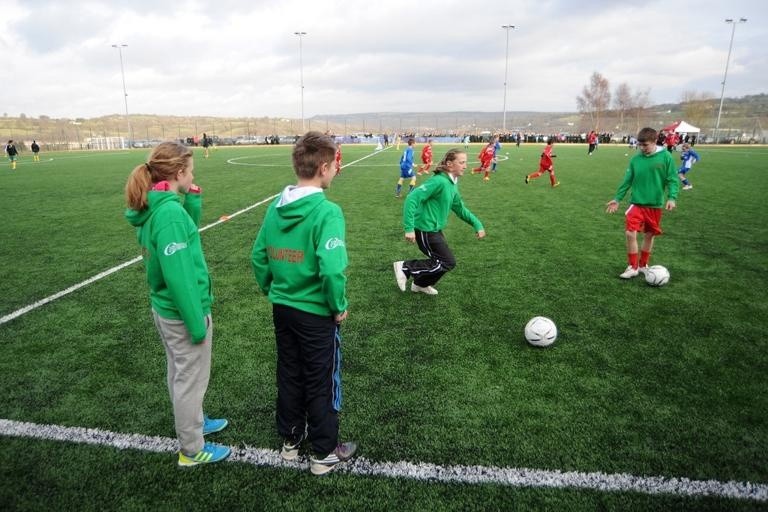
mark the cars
[723,132,758,145]
[132,132,376,148]
[609,134,637,144]
[688,135,713,143]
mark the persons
[392,148,485,295]
[622,129,701,190]
[605,127,681,279]
[31,140,41,163]
[461,130,615,188]
[189,133,213,158]
[250,132,357,476]
[5,140,19,172]
[331,131,373,144]
[264,134,299,144]
[376,130,463,198]
[123,142,232,467]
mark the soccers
[646,264,670,286]
[524,316,558,347]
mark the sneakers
[278,421,312,462]
[395,194,403,198]
[482,177,490,182]
[392,259,410,292]
[682,185,692,190]
[638,263,650,275]
[552,181,561,188]
[202,414,231,438]
[417,169,430,176]
[618,264,640,280]
[471,168,475,175]
[525,175,529,185]
[307,440,359,476]
[410,279,439,296]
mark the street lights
[714,16,748,138]
[111,43,134,139]
[501,23,516,134]
[294,30,307,135]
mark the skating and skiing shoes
[175,440,233,468]
